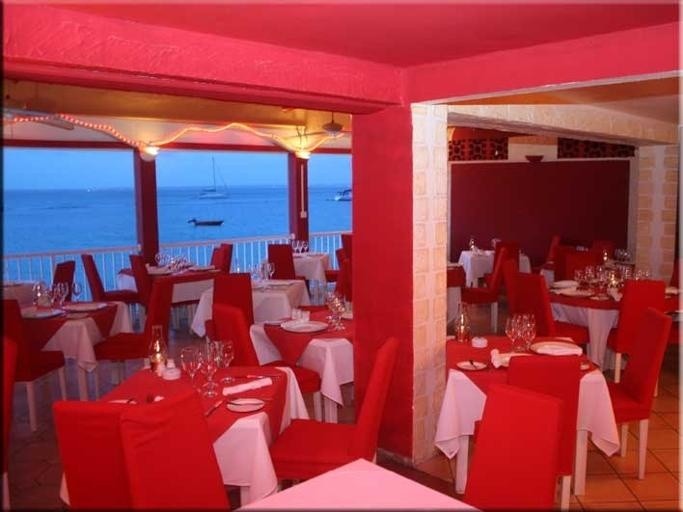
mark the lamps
[294,121,312,160]
[321,111,344,138]
[139,142,161,163]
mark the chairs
[269,337,399,491]
[1,336,18,512]
[463,382,563,511]
[51,399,231,512]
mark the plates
[61,304,107,311]
[227,398,264,412]
[282,321,328,334]
[456,360,487,371]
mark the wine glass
[180,346,202,394]
[250,261,275,284]
[292,240,308,255]
[505,314,536,352]
[155,252,187,274]
[214,341,235,385]
[31,280,69,305]
[198,351,219,398]
[73,283,84,303]
[325,289,348,332]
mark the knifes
[204,400,224,417]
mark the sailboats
[197,154,228,199]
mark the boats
[324,183,352,202]
[186,219,222,225]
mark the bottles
[574,248,650,303]
[454,303,472,343]
[148,324,168,372]
